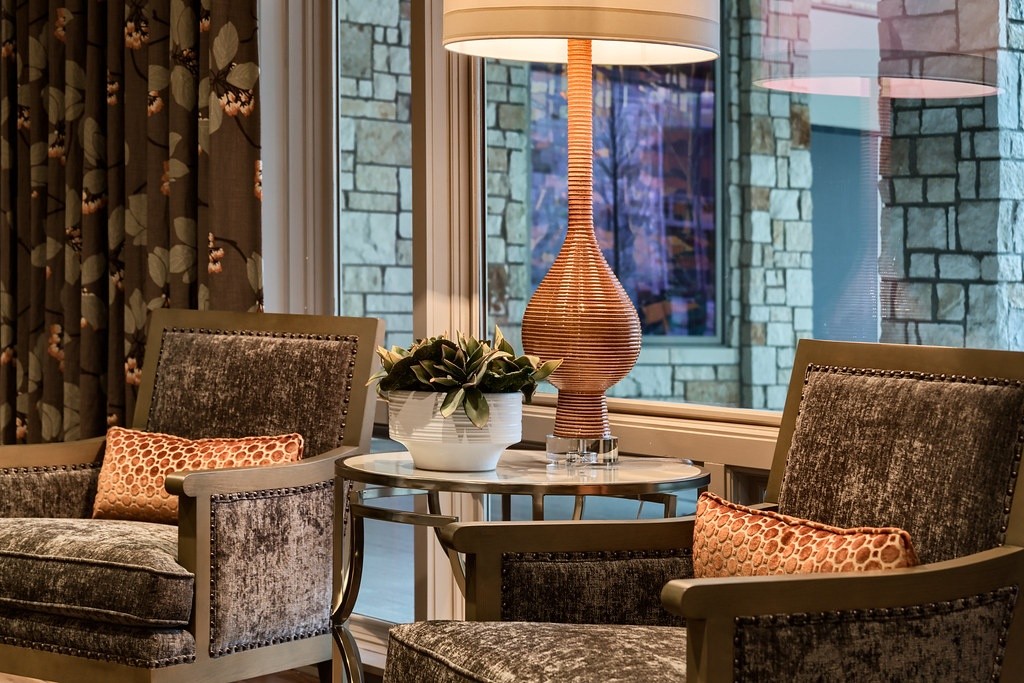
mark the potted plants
[378,320,565,473]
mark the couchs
[0,303,384,682]
[379,338,1024,682]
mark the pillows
[688,491,922,584]
[89,427,306,525]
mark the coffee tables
[326,447,714,626]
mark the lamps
[442,0,724,463]
